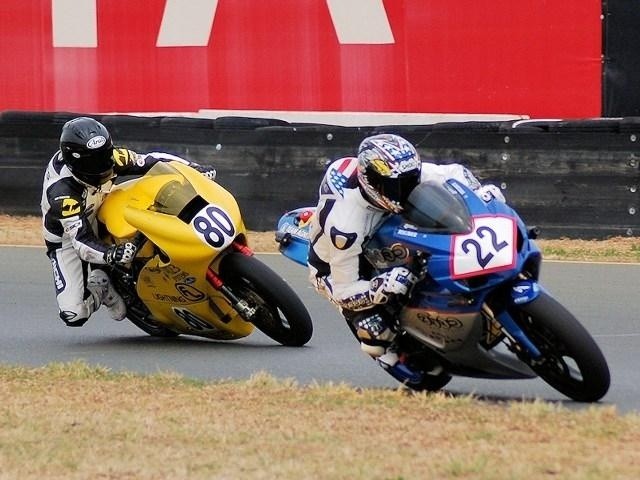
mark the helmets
[356,132,422,215]
[60,116,117,188]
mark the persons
[307,134,481,384]
[40,117,216,326]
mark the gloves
[103,242,138,266]
[473,182,506,206]
[368,265,419,310]
[194,164,216,180]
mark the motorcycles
[90,159,313,347]
[275,178,610,402]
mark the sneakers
[88,268,127,321]
[379,348,424,385]
